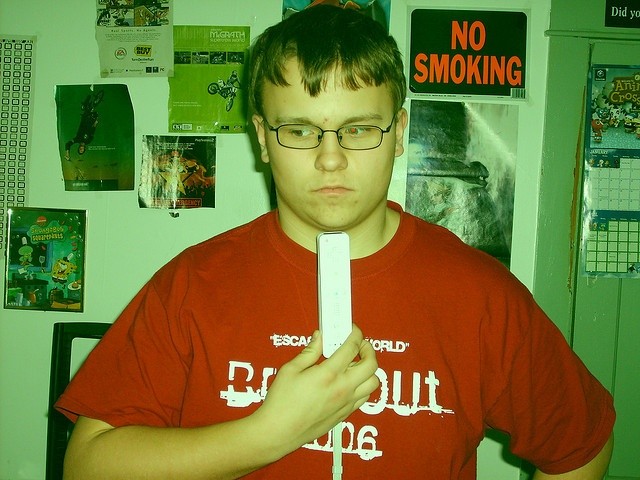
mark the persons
[219,71,243,94]
[64,108,100,161]
[53,5,617,480]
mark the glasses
[262,114,396,150]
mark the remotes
[316,230,353,359]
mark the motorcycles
[208,76,242,112]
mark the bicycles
[78,89,105,123]
